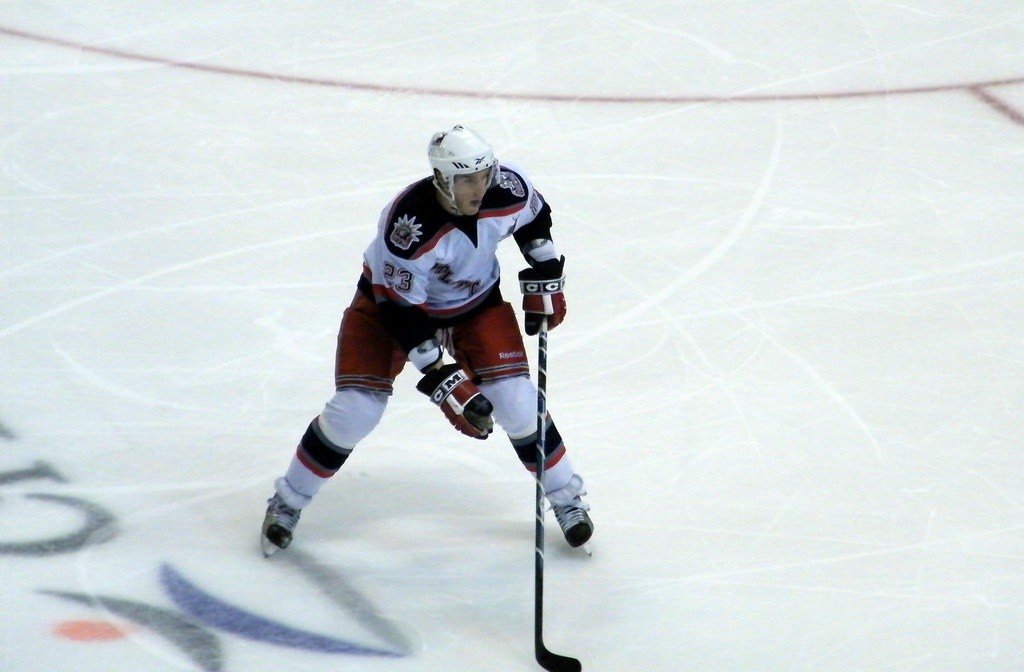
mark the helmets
[428,125,494,187]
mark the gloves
[519,254,567,337]
[416,363,493,441]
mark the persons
[261,124,594,562]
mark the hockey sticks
[530,319,584,672]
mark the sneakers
[260,494,302,558]
[552,494,594,557]
